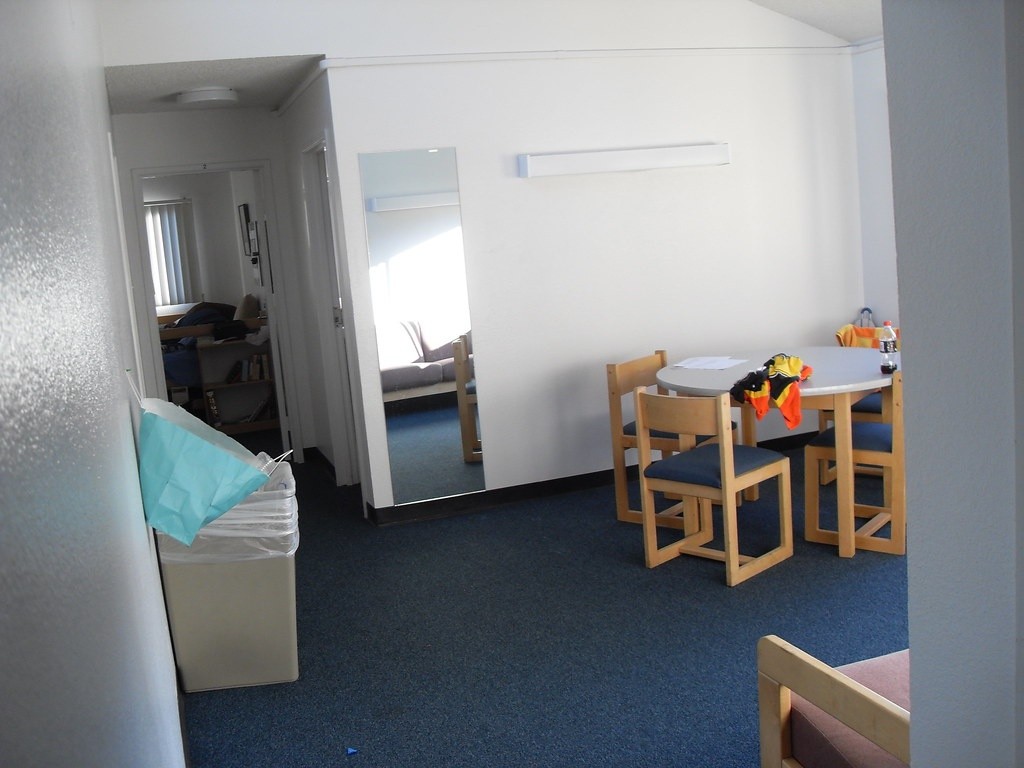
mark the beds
[157,313,267,409]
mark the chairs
[756,633,910,768]
[632,385,795,590]
[805,369,906,556]
[818,386,893,484]
[606,350,743,532]
[452,333,483,463]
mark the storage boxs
[153,461,301,692]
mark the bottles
[879,321,897,373]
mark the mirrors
[357,146,488,504]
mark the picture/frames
[238,203,252,256]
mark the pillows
[234,294,258,320]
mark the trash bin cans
[154,461,299,694]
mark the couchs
[375,313,476,417]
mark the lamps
[174,88,240,110]
[517,141,731,181]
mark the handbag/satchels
[125,365,294,545]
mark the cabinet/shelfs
[196,339,279,434]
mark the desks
[656,344,902,502]
